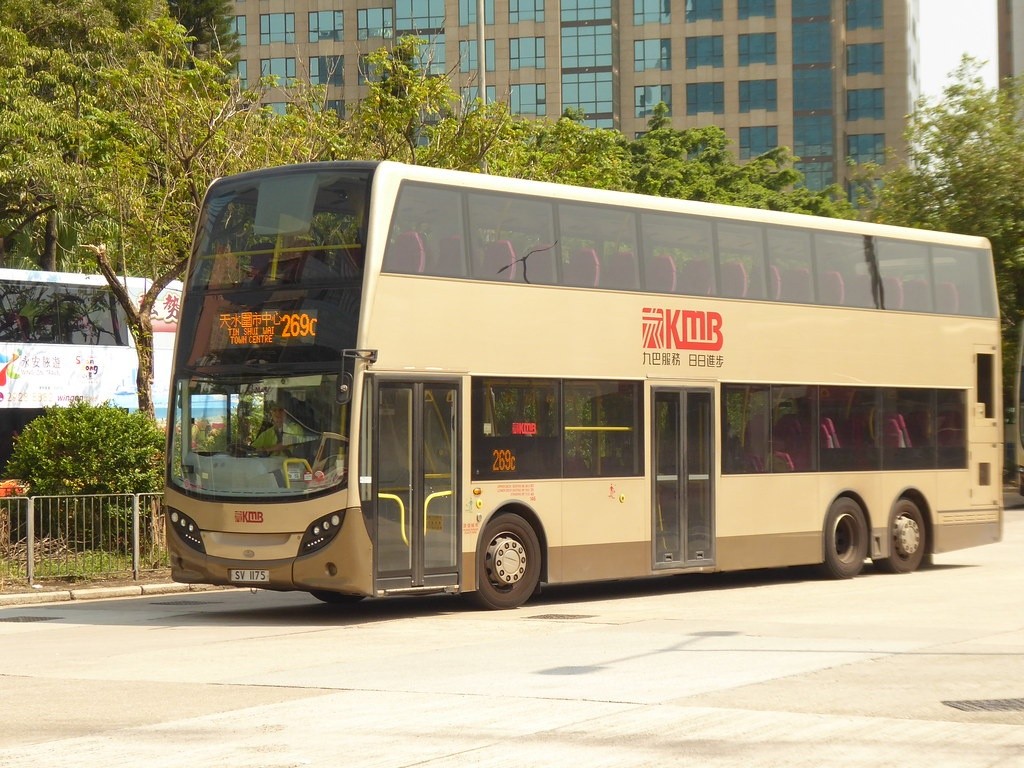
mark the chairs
[733,403,966,475]
[236,231,961,316]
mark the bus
[161,159,1005,609]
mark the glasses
[270,408,284,412]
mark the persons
[247,397,305,460]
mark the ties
[276,432,283,444]
